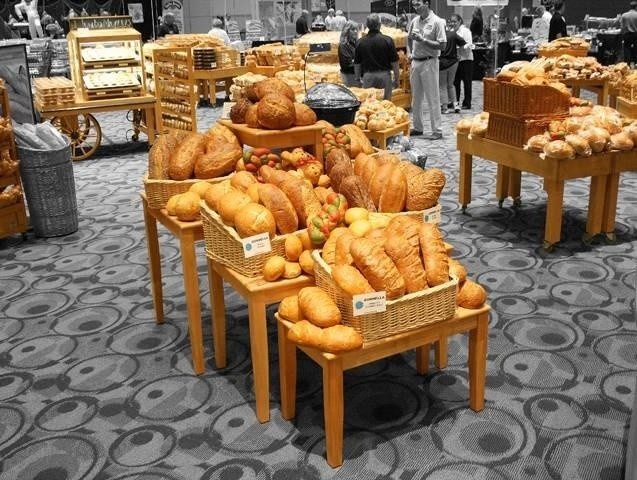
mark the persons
[437,17,474,114]
[622,1,636,65]
[447,14,473,110]
[470,6,484,40]
[404,0,447,142]
[325,8,347,31]
[296,10,311,35]
[548,2,567,42]
[205,18,245,54]
[155,12,180,40]
[338,20,362,88]
[354,13,400,101]
[482,6,551,67]
[13,0,45,39]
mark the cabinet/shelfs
[385,59,413,107]
[0,86,28,239]
[149,46,198,137]
[68,26,146,106]
[196,65,286,105]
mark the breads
[520,96,637,160]
[166,183,215,224]
[0,117,22,209]
[321,218,450,305]
[147,124,242,182]
[141,23,410,128]
[446,258,486,309]
[455,110,490,138]
[230,77,317,129]
[239,120,375,281]
[499,35,637,101]
[276,286,364,351]
[355,98,410,132]
[322,148,447,211]
[206,166,321,240]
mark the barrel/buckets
[595,33,622,65]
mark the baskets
[141,170,237,209]
[482,77,572,148]
[371,201,442,227]
[310,247,459,343]
[199,199,309,278]
[538,48,588,57]
[15,133,78,238]
[349,144,385,170]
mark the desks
[508,145,635,231]
[564,75,610,106]
[362,118,411,152]
[271,303,493,468]
[134,191,206,379]
[451,123,611,250]
[220,113,327,173]
[202,253,312,427]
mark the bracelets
[420,38,427,44]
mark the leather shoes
[428,133,442,140]
[410,129,423,135]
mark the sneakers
[442,102,470,114]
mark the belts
[413,56,433,61]
